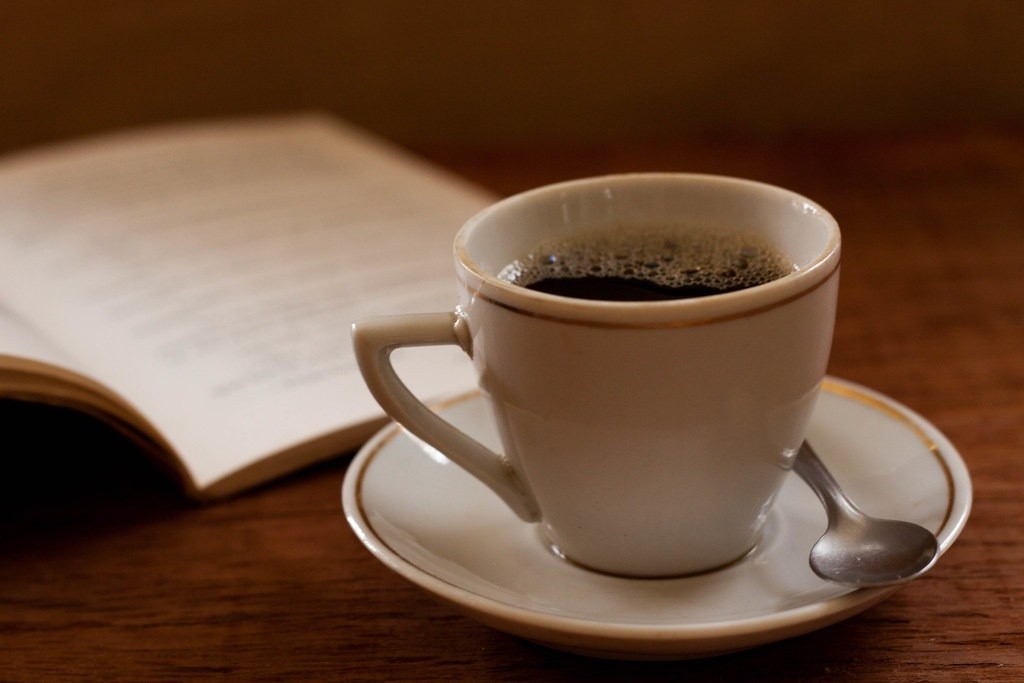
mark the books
[1,112,512,507]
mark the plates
[340,377,971,665]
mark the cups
[351,173,840,577]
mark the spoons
[791,440,939,587]
